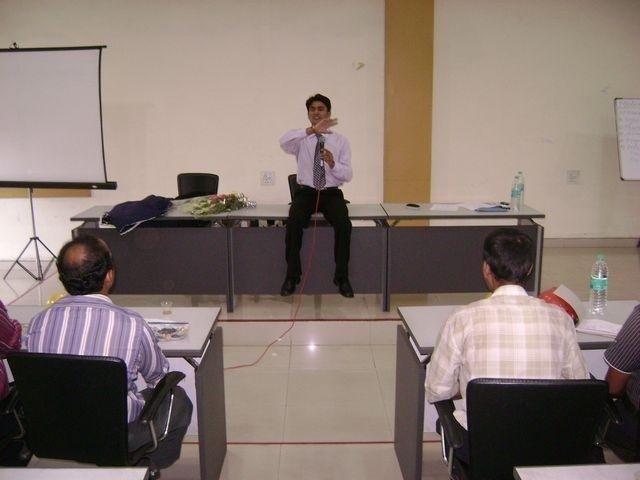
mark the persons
[602,304,639,410]
[0,301,22,399]
[278,93,355,297]
[424,227,587,465]
[25,235,193,480]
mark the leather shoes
[149,468,161,480]
[280,274,300,296]
[333,276,355,297]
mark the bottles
[588,254,609,315]
[517,171,524,210]
[510,176,521,212]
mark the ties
[312,132,326,190]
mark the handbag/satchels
[0,380,33,467]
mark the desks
[394,300,640,480]
[232,203,388,311]
[3,307,226,480]
[379,203,545,312]
[70,205,232,312]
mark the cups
[161,300,173,314]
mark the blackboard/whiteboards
[614,98,640,181]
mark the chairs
[7,352,141,467]
[424,364,623,480]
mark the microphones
[319,136,326,166]
